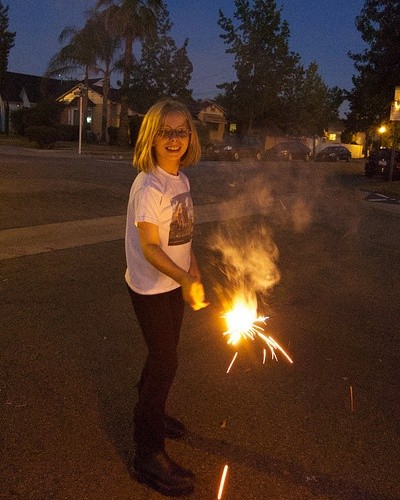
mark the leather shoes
[133,411,186,441]
[128,450,197,498]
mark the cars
[316,145,351,163]
[262,142,311,162]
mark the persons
[127,99,211,492]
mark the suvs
[206,135,262,162]
[364,148,400,182]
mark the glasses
[158,126,192,138]
